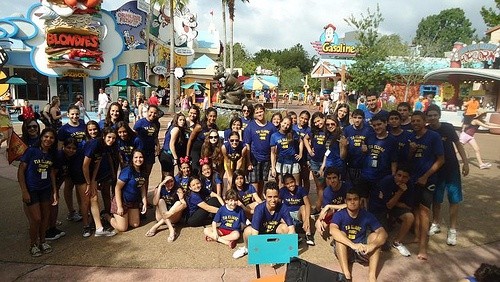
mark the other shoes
[480,164,491,170]
[310,207,321,215]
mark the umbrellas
[106,78,147,88]
[242,74,278,91]
[181,81,209,105]
[135,79,159,98]
[0,73,41,107]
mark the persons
[0,89,500,282]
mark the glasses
[243,109,250,113]
[326,123,335,126]
[209,135,218,139]
[230,139,239,142]
[27,125,38,130]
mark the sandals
[30,245,41,257]
[39,242,52,254]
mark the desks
[440,110,491,132]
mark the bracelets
[86,183,90,185]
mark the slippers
[230,241,236,248]
[145,231,156,237]
[167,231,177,242]
[206,237,214,242]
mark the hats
[475,109,488,119]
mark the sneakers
[447,228,457,246]
[427,224,441,236]
[67,202,153,238]
[298,236,303,241]
[392,240,411,256]
[56,220,61,225]
[232,246,248,258]
[305,234,314,246]
[44,231,60,241]
[49,227,65,237]
